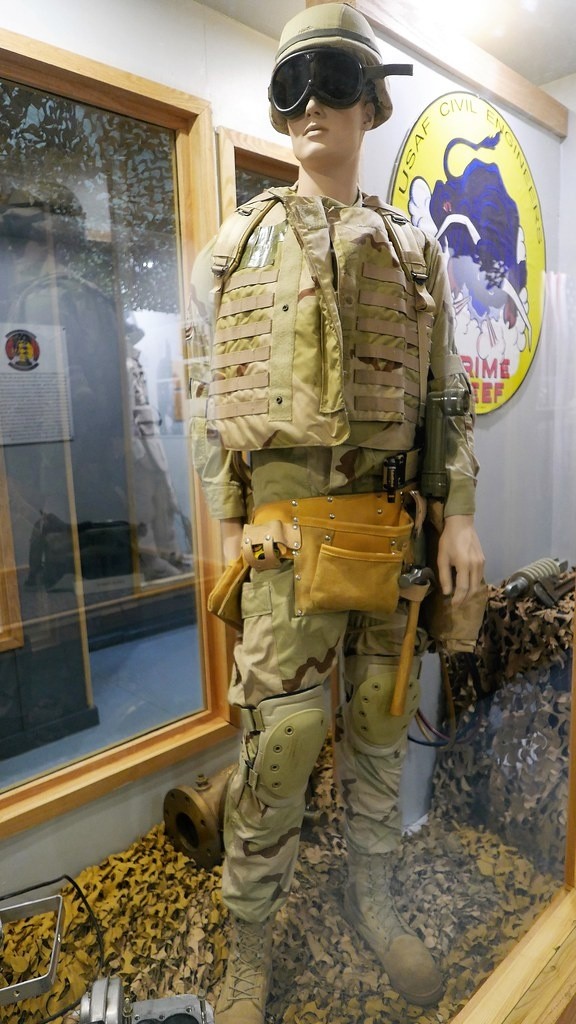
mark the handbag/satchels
[27,513,138,592]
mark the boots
[344,832,443,1005]
[214,909,273,1024]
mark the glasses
[267,48,413,118]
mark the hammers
[389,566,437,716]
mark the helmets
[269,3,393,135]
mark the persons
[184,3,485,1024]
[1,181,190,678]
